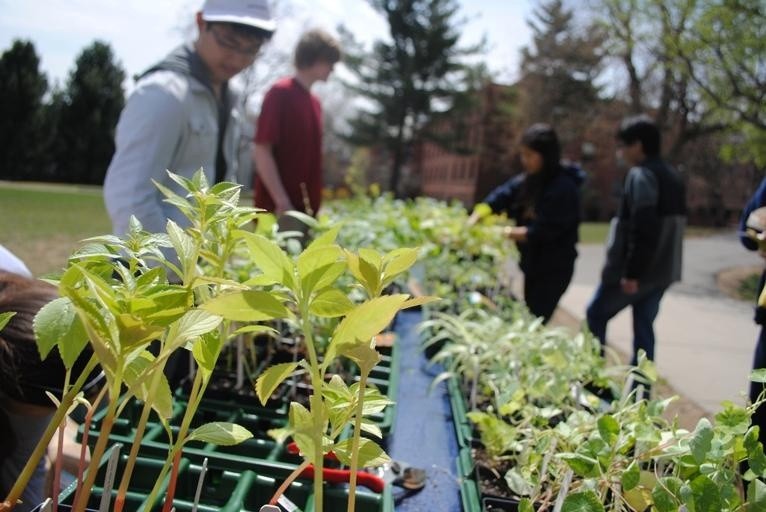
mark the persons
[589,114,684,403]
[101,0,296,292]
[469,123,585,328]
[252,30,341,230]
[738,172,766,501]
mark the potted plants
[20,164,766,510]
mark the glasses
[211,27,264,54]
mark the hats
[202,0,276,38]
